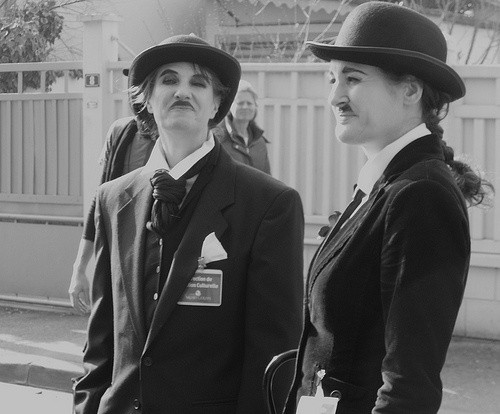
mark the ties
[150,144,220,236]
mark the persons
[71,34,304,414]
[67,120,157,317]
[282,0,492,414]
[453,373,456,376]
[213,89,271,176]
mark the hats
[305,1,465,102]
[127,35,242,129]
